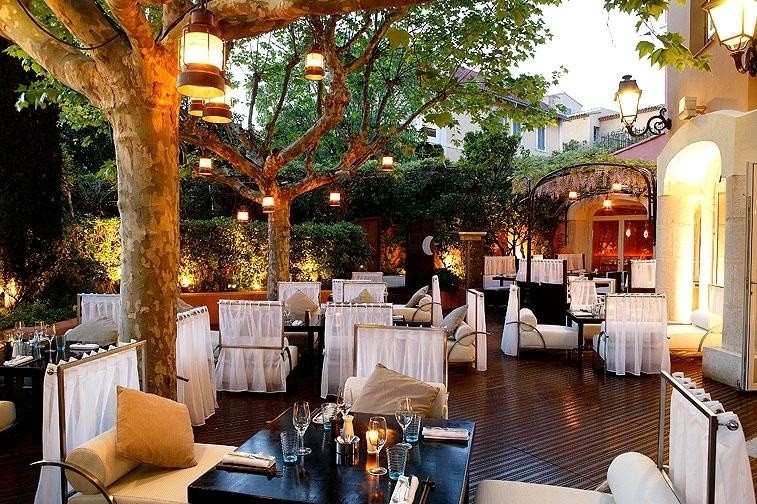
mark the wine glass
[337,384,353,416]
[282,301,291,326]
[292,401,312,455]
[45,324,56,363]
[33,321,44,359]
[395,397,413,449]
[368,416,388,476]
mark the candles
[366,429,378,453]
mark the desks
[283,313,325,353]
[566,310,606,360]
[0,340,117,394]
[577,276,616,294]
[188,404,476,504]
[492,276,515,287]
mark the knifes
[434,428,465,432]
[228,452,273,461]
[16,356,26,361]
[403,474,412,501]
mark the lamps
[199,157,213,175]
[305,39,325,81]
[177,0,227,97]
[236,205,248,221]
[568,191,577,199]
[329,187,341,207]
[613,74,672,137]
[201,98,233,125]
[262,188,275,213]
[612,183,622,191]
[185,97,206,114]
[700,0,757,80]
[382,152,394,170]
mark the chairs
[76,293,121,330]
[430,288,491,376]
[596,271,622,294]
[177,297,220,358]
[1,399,16,431]
[320,300,393,401]
[499,284,585,361]
[591,291,673,379]
[570,281,597,308]
[331,279,371,303]
[393,274,444,327]
[351,272,383,282]
[175,306,219,427]
[342,282,388,304]
[474,368,757,504]
[30,339,239,504]
[667,283,725,351]
[277,281,322,311]
[211,298,299,394]
[343,322,448,422]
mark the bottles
[343,414,354,441]
[4,340,13,361]
[12,342,19,357]
[20,338,30,356]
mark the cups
[405,412,422,443]
[336,435,361,466]
[56,335,66,350]
[386,442,408,480]
[4,321,29,360]
[592,303,601,319]
[280,431,299,464]
[321,403,338,430]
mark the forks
[392,475,407,503]
[9,355,21,364]
[425,424,442,429]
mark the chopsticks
[419,475,434,504]
[310,408,320,418]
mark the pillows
[404,285,430,308]
[351,289,377,303]
[115,384,199,469]
[351,362,440,419]
[439,305,470,337]
[284,290,319,322]
[64,316,119,348]
